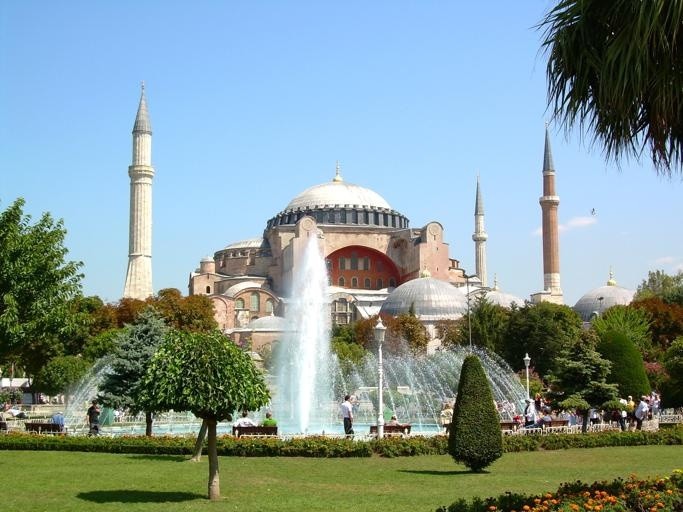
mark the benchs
[231,426,277,436]
[499,419,569,431]
[370,425,411,435]
[24,422,63,434]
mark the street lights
[462,273,479,353]
[372,316,386,438]
[523,352,532,398]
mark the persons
[493,393,660,434]
[386,415,411,437]
[234,411,277,438]
[87,400,100,437]
[52,411,64,431]
[441,404,453,435]
[340,395,354,441]
